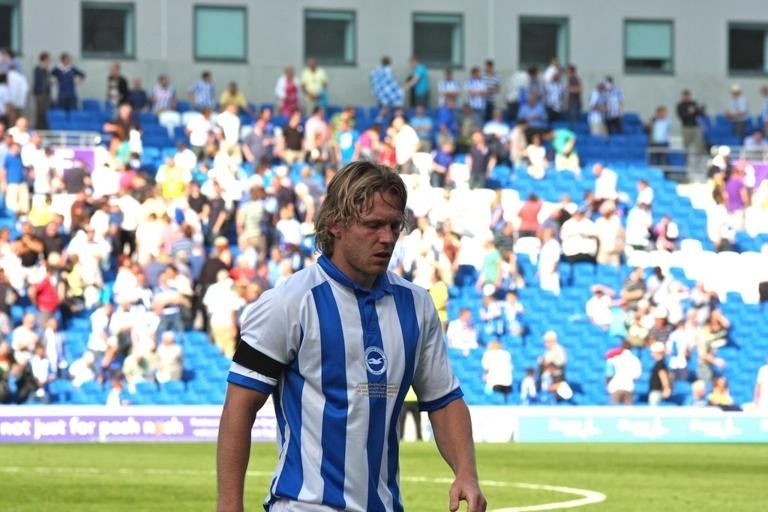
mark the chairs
[0,99,767,407]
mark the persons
[0,46,767,404]
[216,161,487,512]
